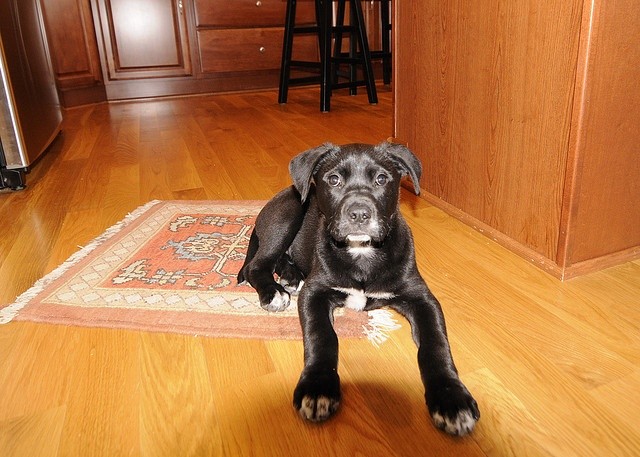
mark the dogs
[237,143,480,437]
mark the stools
[279,1,379,112]
[334,0,390,94]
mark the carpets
[1,199,402,349]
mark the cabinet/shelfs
[39,0,104,90]
[90,0,392,101]
